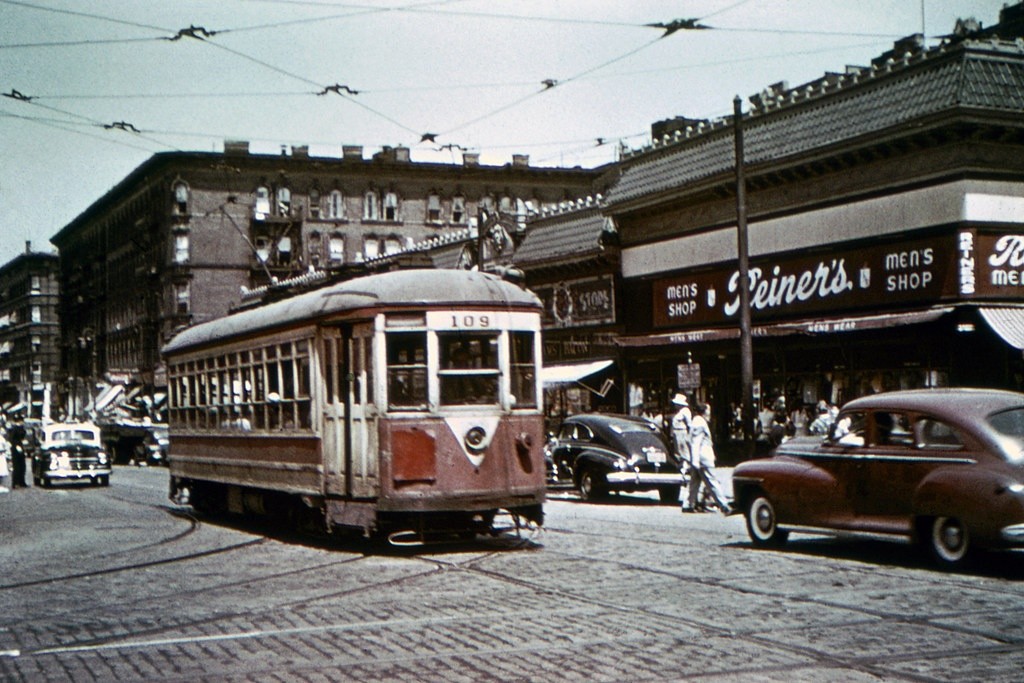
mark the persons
[0,414,10,493]
[11,416,29,488]
[839,411,895,447]
[809,400,834,434]
[670,394,692,473]
[681,403,732,513]
[782,396,809,443]
[757,399,775,434]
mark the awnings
[542,360,613,382]
[978,307,1024,349]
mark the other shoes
[725,511,734,516]
[682,507,697,513]
[696,505,714,514]
[0,486,9,494]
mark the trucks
[99,419,169,465]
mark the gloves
[21,440,29,444]
[17,446,22,452]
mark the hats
[13,412,24,422]
[672,394,689,406]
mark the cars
[30,422,112,489]
[729,389,1023,567]
[545,414,694,507]
[23,417,44,457]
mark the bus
[161,263,547,549]
